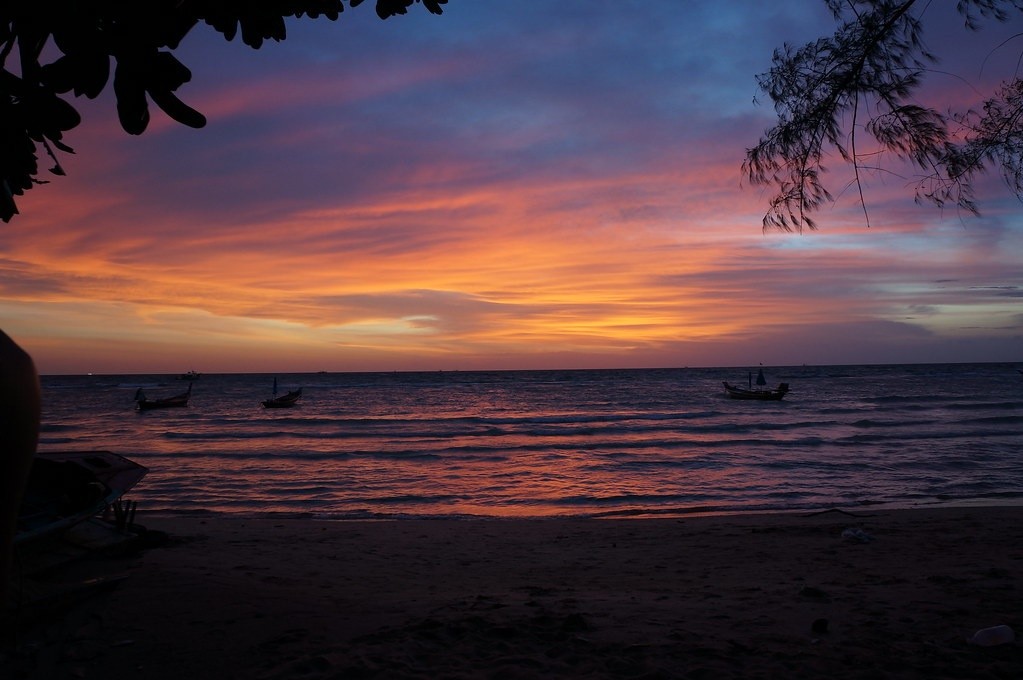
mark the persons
[134,387,148,401]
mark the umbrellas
[273,377,278,400]
[756,370,766,392]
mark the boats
[721,370,794,403]
[133,383,195,411]
[259,377,304,410]
